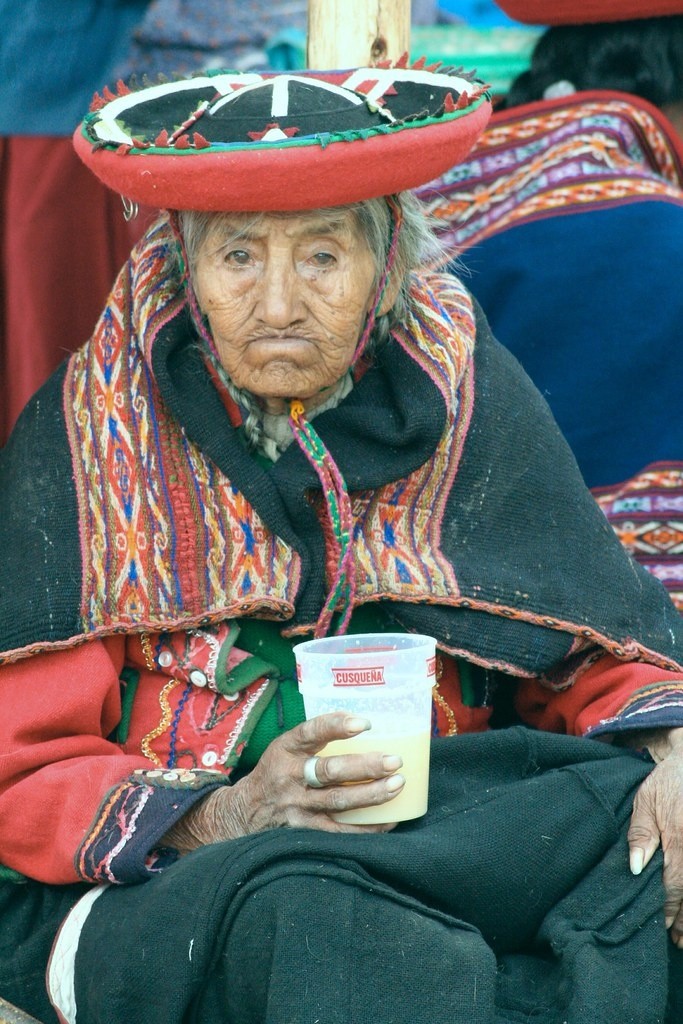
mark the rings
[304,755,326,788]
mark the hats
[72,69,493,214]
[494,2,681,25]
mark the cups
[291,631,440,822]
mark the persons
[390,1,683,617]
[1,63,683,1023]
[4,0,315,443]
